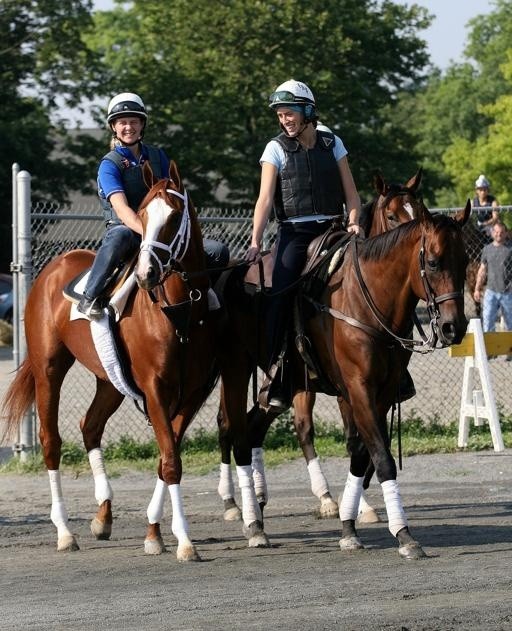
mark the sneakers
[77,294,103,315]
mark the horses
[251,166,432,524]
[203,197,471,560]
[462,216,512,323]
[0,160,224,562]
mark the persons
[241,79,366,409]
[77,93,229,319]
[471,175,502,238]
[474,222,512,361]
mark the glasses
[109,101,145,115]
[269,91,297,102]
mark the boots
[268,351,290,408]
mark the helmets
[107,92,148,122]
[268,79,316,110]
[476,178,491,188]
